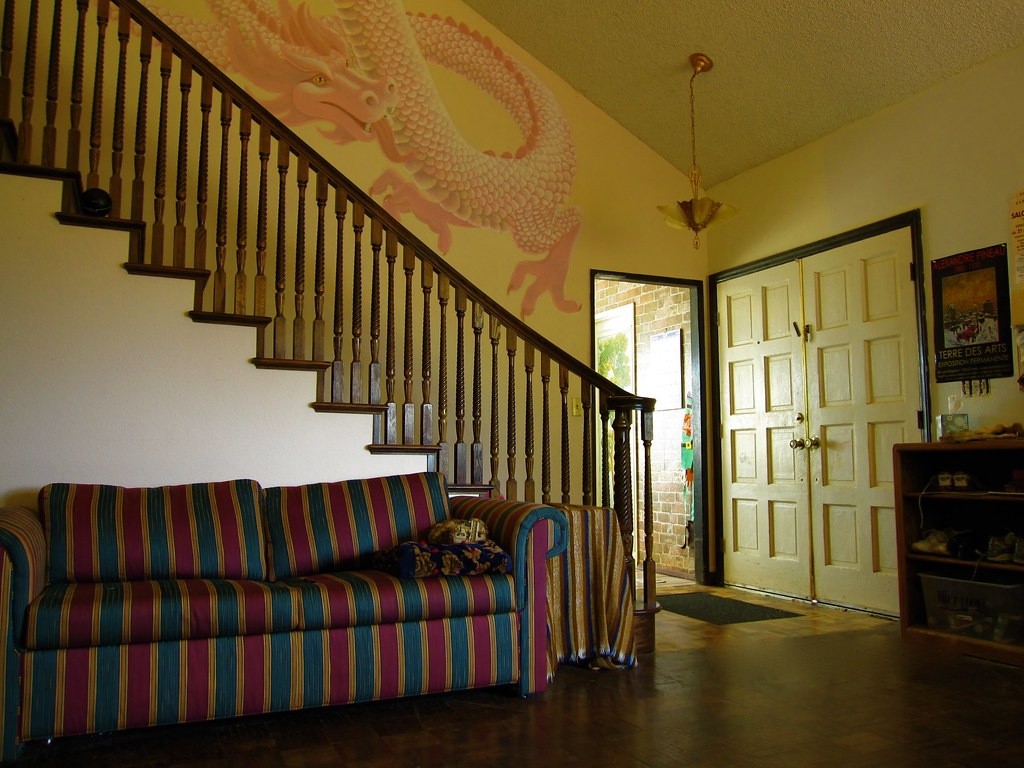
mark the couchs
[0,473,569,768]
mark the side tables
[546,504,638,680]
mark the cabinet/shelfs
[891,442,1024,666]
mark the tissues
[935,394,969,443]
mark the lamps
[656,52,743,251]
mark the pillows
[388,540,513,575]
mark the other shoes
[912,526,975,555]
[1014,539,1024,564]
[937,471,952,491]
[987,533,1012,564]
[925,602,1023,645]
[953,471,971,490]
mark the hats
[977,314,984,319]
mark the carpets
[656,591,806,625]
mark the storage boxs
[914,572,1023,644]
